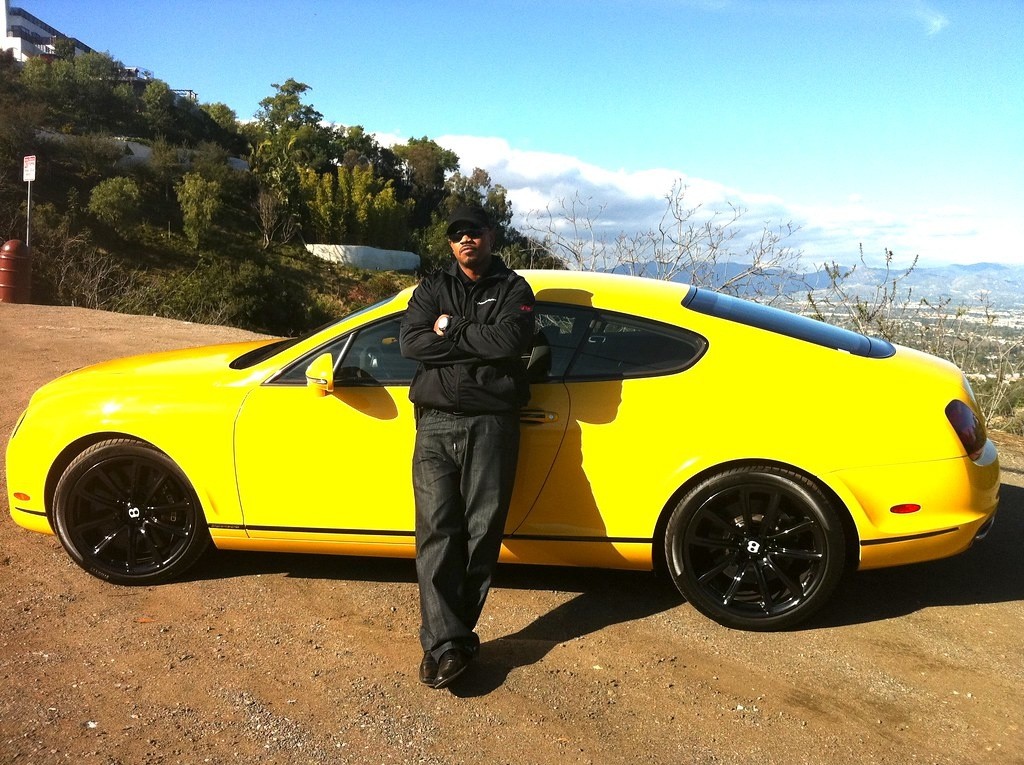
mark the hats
[447,206,491,233]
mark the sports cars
[5,271,999,631]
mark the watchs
[439,316,452,336]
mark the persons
[400,206,536,688]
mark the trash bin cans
[1,238,32,304]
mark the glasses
[449,225,490,242]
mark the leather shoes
[419,648,438,683]
[435,633,478,688]
